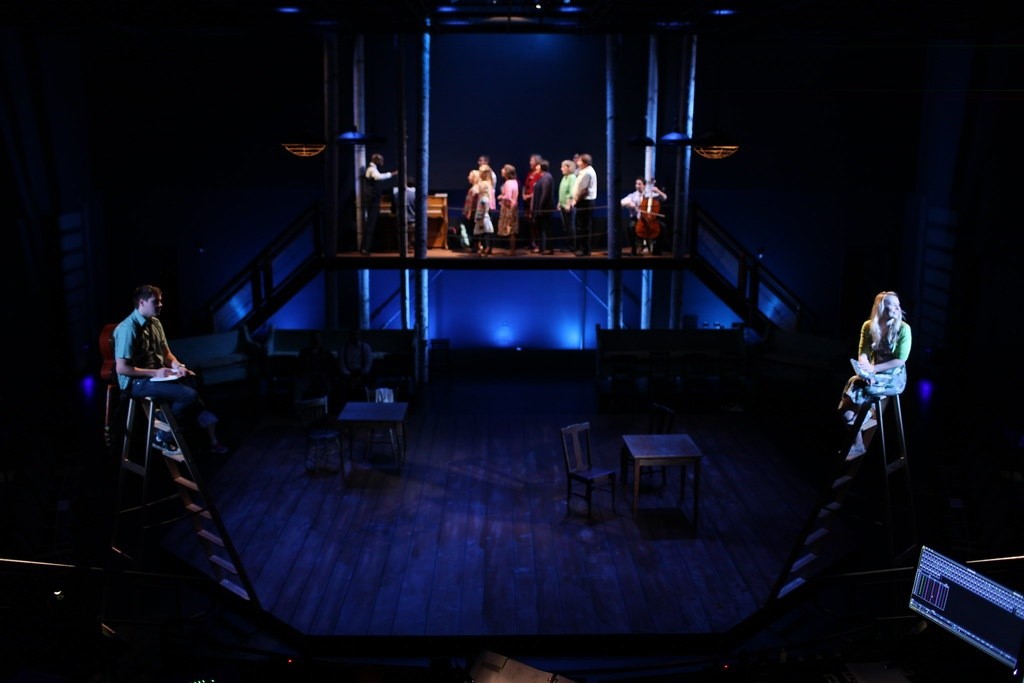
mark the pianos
[379,192,451,250]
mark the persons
[114,285,226,456]
[838,291,913,459]
[393,178,416,253]
[360,154,398,253]
[464,154,597,256]
[621,178,667,254]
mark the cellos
[634,177,662,241]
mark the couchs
[762,328,844,401]
[273,331,417,411]
[594,329,746,415]
[171,325,268,399]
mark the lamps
[281,143,326,158]
[693,144,740,160]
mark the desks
[621,434,702,521]
[380,193,448,250]
[337,402,409,479]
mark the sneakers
[151,435,182,455]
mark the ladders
[762,390,931,608]
[97,393,267,648]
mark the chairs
[364,385,407,462]
[296,396,342,475]
[561,422,616,520]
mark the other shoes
[531,250,541,253]
[210,444,228,453]
[576,250,592,255]
[544,251,554,255]
